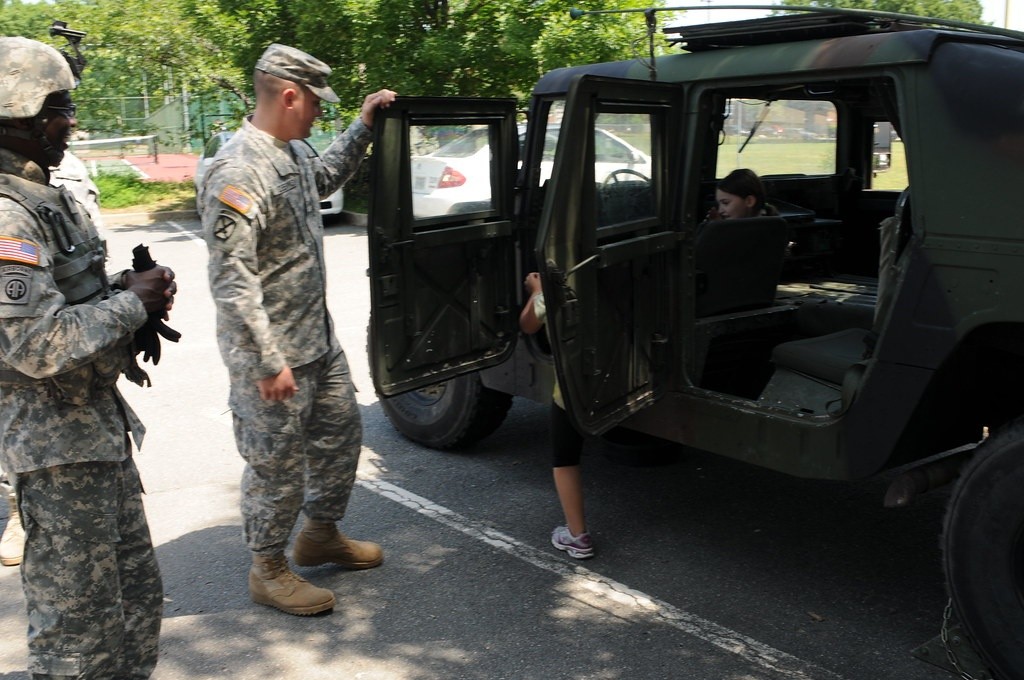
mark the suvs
[365,5,1024,680]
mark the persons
[715,168,779,219]
[0,148,107,567]
[519,272,594,559]
[197,43,397,615]
[0,35,181,680]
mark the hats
[254,42,341,104]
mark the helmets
[0,37,76,118]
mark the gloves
[131,243,182,365]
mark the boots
[291,516,383,569]
[0,497,27,565]
[248,551,335,614]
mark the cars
[740,125,817,139]
[412,123,649,219]
[194,129,344,225]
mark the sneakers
[551,526,593,557]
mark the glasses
[48,105,76,117]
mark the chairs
[694,215,789,318]
[770,185,913,386]
